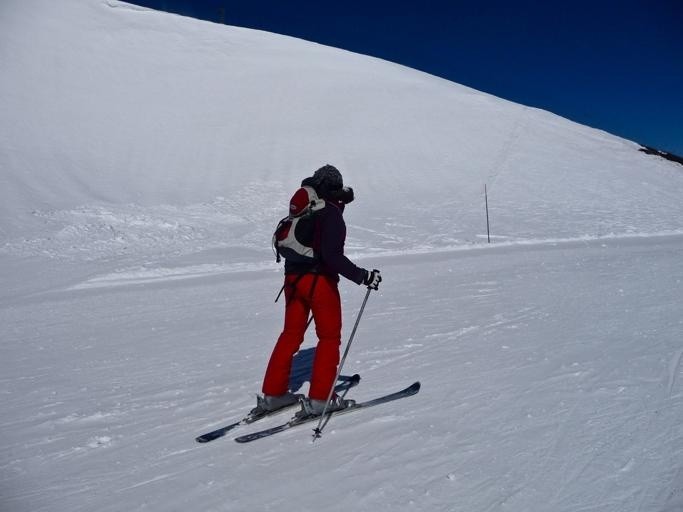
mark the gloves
[361,271,382,290]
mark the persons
[258,164,382,414]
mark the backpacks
[272,186,326,263]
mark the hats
[301,164,354,204]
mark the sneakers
[310,396,349,415]
[264,391,297,411]
